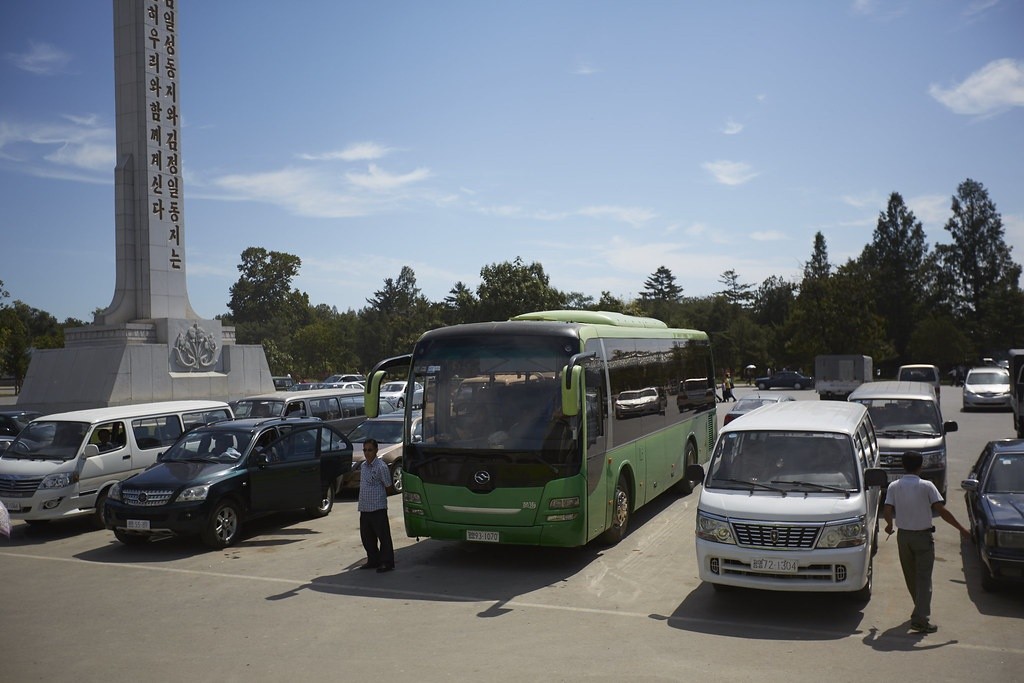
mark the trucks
[814,354,881,401]
[983,347,1024,439]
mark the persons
[714,372,737,403]
[358,439,395,573]
[745,368,752,386]
[884,451,977,634]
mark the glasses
[363,448,373,452]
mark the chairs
[62,417,182,449]
[210,434,233,455]
[252,399,333,420]
[254,435,309,460]
[465,378,559,435]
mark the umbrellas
[747,365,756,370]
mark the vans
[895,364,943,407]
[0,400,239,528]
[847,379,959,506]
[685,399,888,604]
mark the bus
[362,309,720,548]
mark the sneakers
[360,561,376,569]
[911,621,938,632]
[376,563,392,572]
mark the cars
[754,371,813,390]
[959,437,1024,596]
[614,386,667,420]
[206,373,538,495]
[723,392,797,442]
[0,410,57,456]
[960,360,1012,411]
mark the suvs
[102,414,355,552]
[676,378,715,413]
